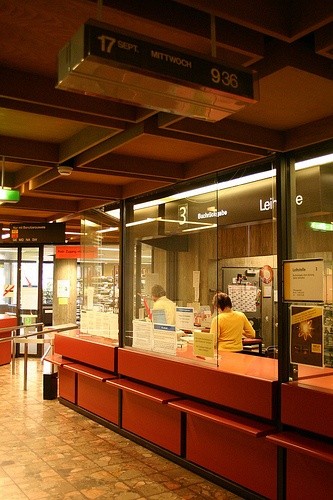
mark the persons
[148,285,178,326]
[210,292,257,353]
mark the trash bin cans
[42,371,59,400]
